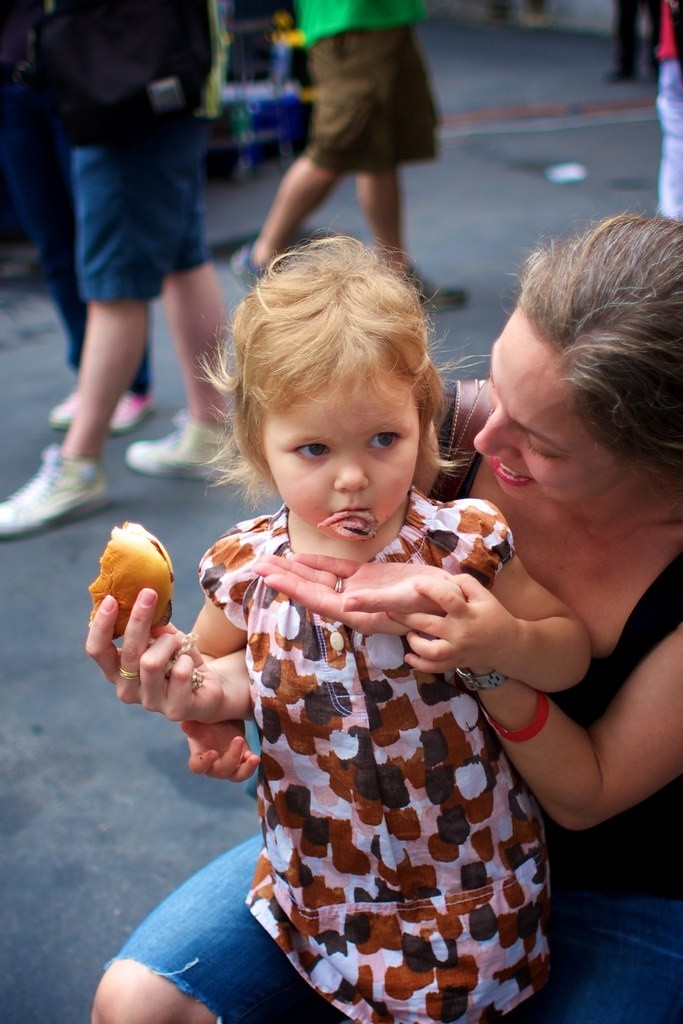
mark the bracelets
[487,691,549,742]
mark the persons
[0,0,235,541]
[227,2,470,310]
[604,0,683,219]
[85,213,683,1024]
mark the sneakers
[0,447,109,539]
[125,413,226,481]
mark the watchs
[455,667,509,691]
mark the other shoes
[230,247,261,288]
[414,282,468,311]
[110,390,157,436]
[49,391,79,431]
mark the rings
[119,666,140,680]
[335,577,341,593]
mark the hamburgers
[88,521,176,640]
